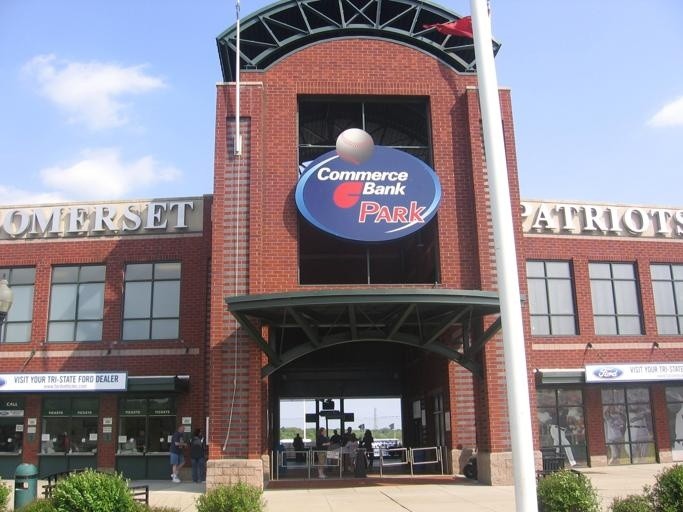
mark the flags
[422,14,473,38]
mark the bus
[356,438,400,459]
[279,438,316,461]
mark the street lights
[0,277,13,327]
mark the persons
[314,428,330,478]
[0,428,152,454]
[169,424,188,484]
[534,388,682,468]
[357,423,374,467]
[292,433,303,462]
[187,429,207,483]
[331,429,340,443]
[350,432,354,439]
[341,427,354,447]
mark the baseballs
[336,128,375,164]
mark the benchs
[42,470,149,510]
[536,469,580,482]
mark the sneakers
[171,473,181,483]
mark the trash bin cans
[14,462,39,511]
